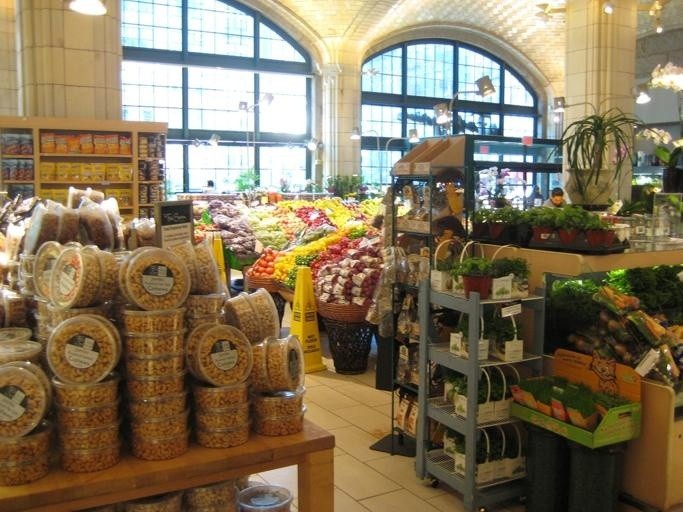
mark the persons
[542,187,567,208]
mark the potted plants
[461,329,489,360]
[550,390,568,422]
[537,389,552,415]
[459,254,492,298]
[532,205,555,245]
[543,107,665,210]
[508,209,532,246]
[450,260,465,294]
[453,378,494,423]
[491,321,524,363]
[488,207,515,240]
[594,392,612,418]
[523,384,538,409]
[431,259,452,293]
[566,399,598,432]
[509,447,527,478]
[474,207,490,239]
[554,202,586,250]
[492,386,511,421]
[511,257,532,298]
[584,214,607,249]
[510,378,522,405]
[444,376,466,402]
[454,440,493,483]
[442,428,459,459]
[449,320,463,356]
[491,256,512,299]
[493,445,510,480]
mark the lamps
[350,127,380,156]
[602,2,615,15]
[385,128,420,163]
[653,11,663,34]
[649,54,683,94]
[552,96,596,115]
[433,103,450,124]
[307,137,323,166]
[599,83,651,115]
[449,75,496,114]
[239,94,272,110]
[192,133,221,147]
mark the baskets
[278,288,296,304]
[314,299,368,323]
[243,273,278,294]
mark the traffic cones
[213,238,227,288]
[289,266,328,375]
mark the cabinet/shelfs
[0,406,337,511]
[470,240,683,512]
[419,269,545,512]
[0,116,164,246]
[371,134,474,453]
